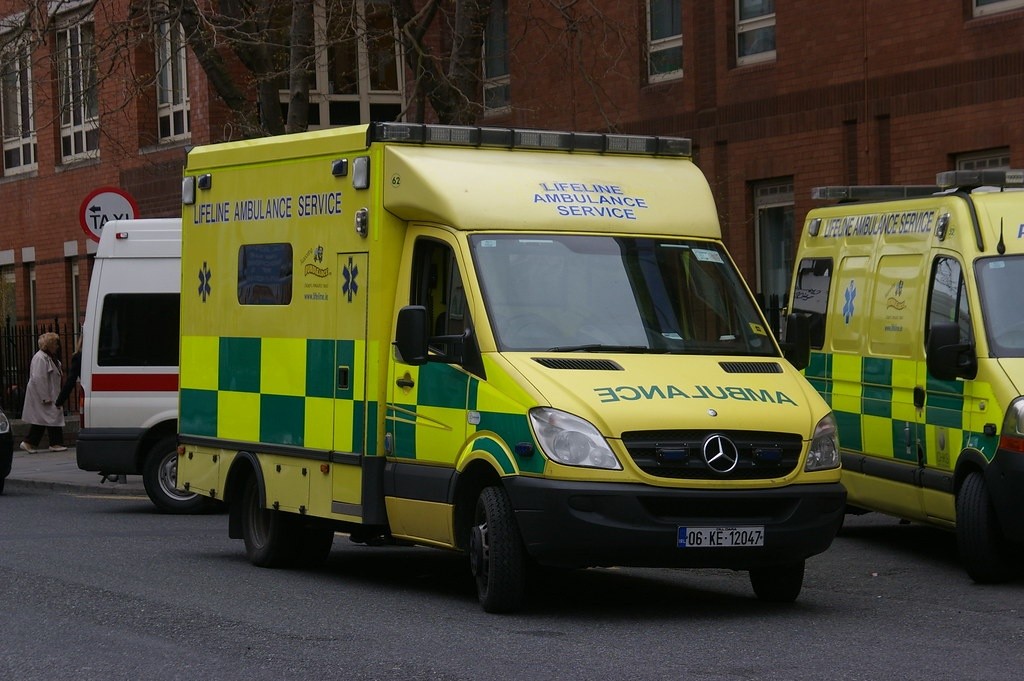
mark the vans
[75,218,213,516]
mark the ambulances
[786,185,1024,587]
[177,122,847,614]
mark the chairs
[434,312,512,354]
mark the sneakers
[20,442,37,454]
[49,446,68,452]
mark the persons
[56,334,83,408]
[20,332,68,454]
[513,270,591,339]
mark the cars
[0,407,14,496]
